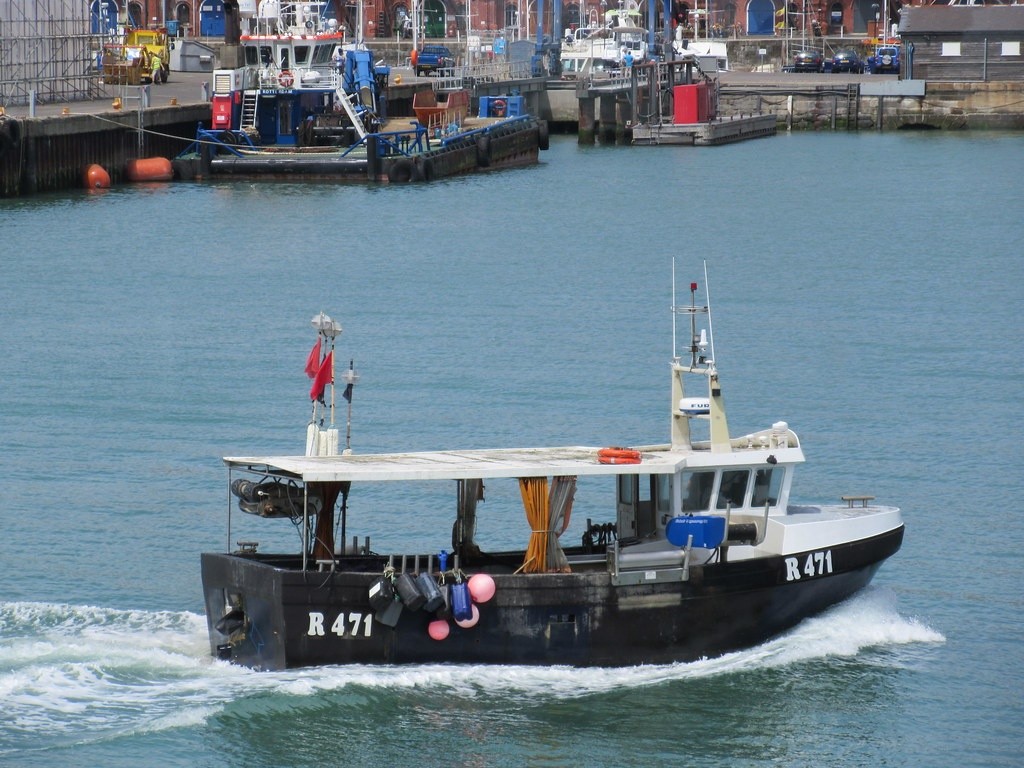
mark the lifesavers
[278,70,295,87]
[387,157,433,183]
[597,446,641,465]
[539,119,551,150]
[478,139,493,167]
[494,100,506,115]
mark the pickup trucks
[414,46,455,77]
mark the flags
[310,352,331,409]
[304,337,321,379]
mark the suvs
[875,46,900,74]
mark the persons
[812,19,828,37]
[620,48,635,78]
[674,22,685,40]
[149,53,165,85]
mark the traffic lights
[674,0,688,23]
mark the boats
[200,257,906,674]
[173,0,549,182]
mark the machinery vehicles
[102,30,170,85]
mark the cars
[831,49,864,73]
[795,51,824,72]
